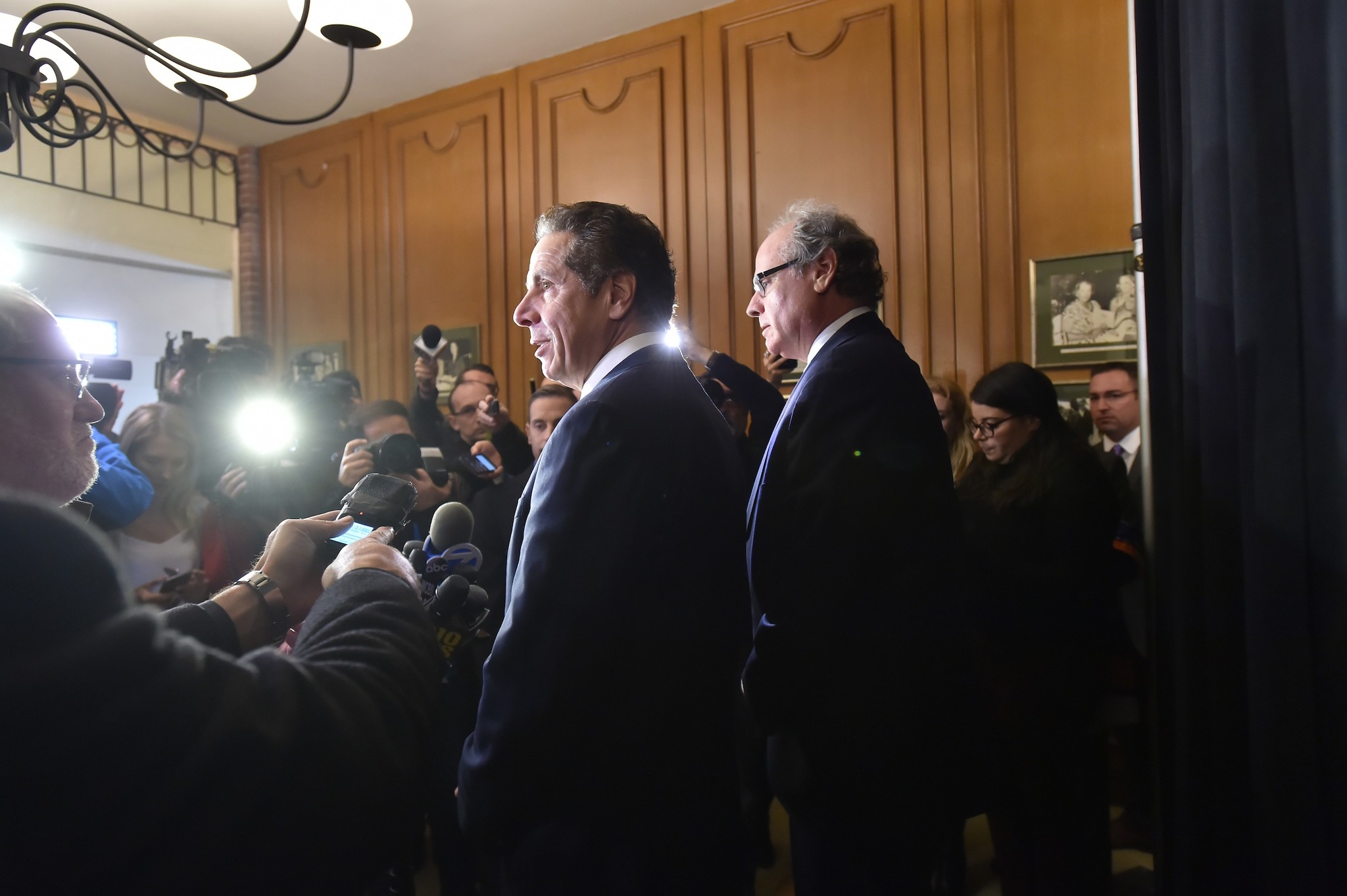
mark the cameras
[353,432,449,488]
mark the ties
[1112,445,1124,457]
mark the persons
[0,198,1144,896]
[1057,280,1119,345]
[1106,274,1137,330]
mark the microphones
[413,324,448,365]
[398,502,489,664]
[89,358,132,380]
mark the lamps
[0,0,415,158]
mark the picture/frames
[779,284,884,387]
[1027,249,1138,369]
[1052,381,1104,447]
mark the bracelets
[233,569,290,651]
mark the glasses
[0,357,91,399]
[454,406,480,416]
[1089,389,1139,404]
[965,414,1015,438]
[753,256,802,297]
